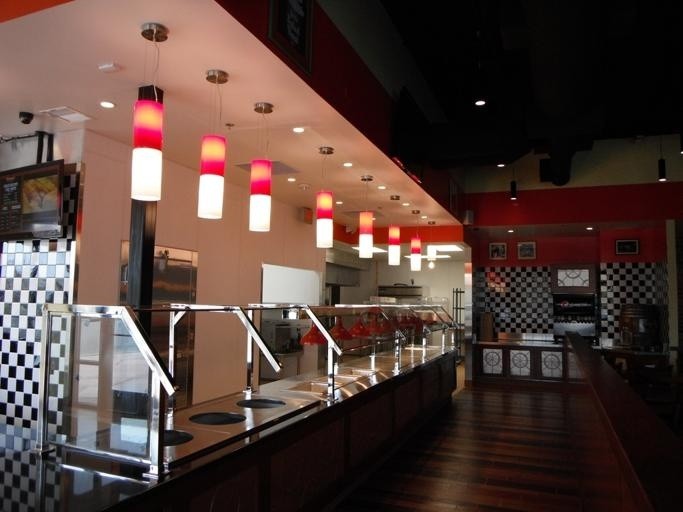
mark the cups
[462,210,475,225]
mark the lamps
[494,142,517,200]
[197,69,229,220]
[130,23,169,202]
[473,82,486,106]
[316,146,436,271]
[249,101,273,234]
[658,127,683,183]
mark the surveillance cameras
[19,112,34,124]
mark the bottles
[593,336,599,344]
[554,316,595,323]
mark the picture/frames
[615,239,639,255]
[266,1,314,78]
[489,242,536,260]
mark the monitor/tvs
[0,159,64,240]
